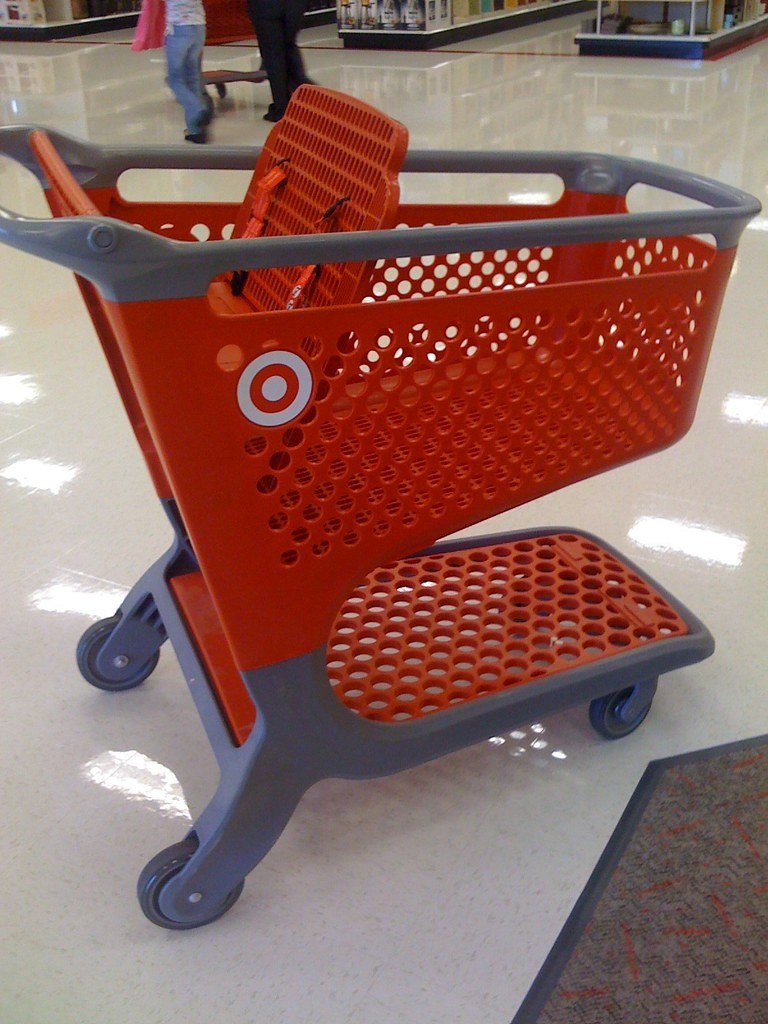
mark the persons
[245,0,317,123]
[164,1,214,144]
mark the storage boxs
[337,0,452,30]
[3,0,53,25]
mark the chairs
[141,82,409,431]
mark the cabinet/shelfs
[0,0,142,41]
[574,0,768,56]
[336,0,610,50]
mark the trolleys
[0,116,765,933]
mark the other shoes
[184,128,205,143]
[264,110,281,122]
[197,112,210,125]
